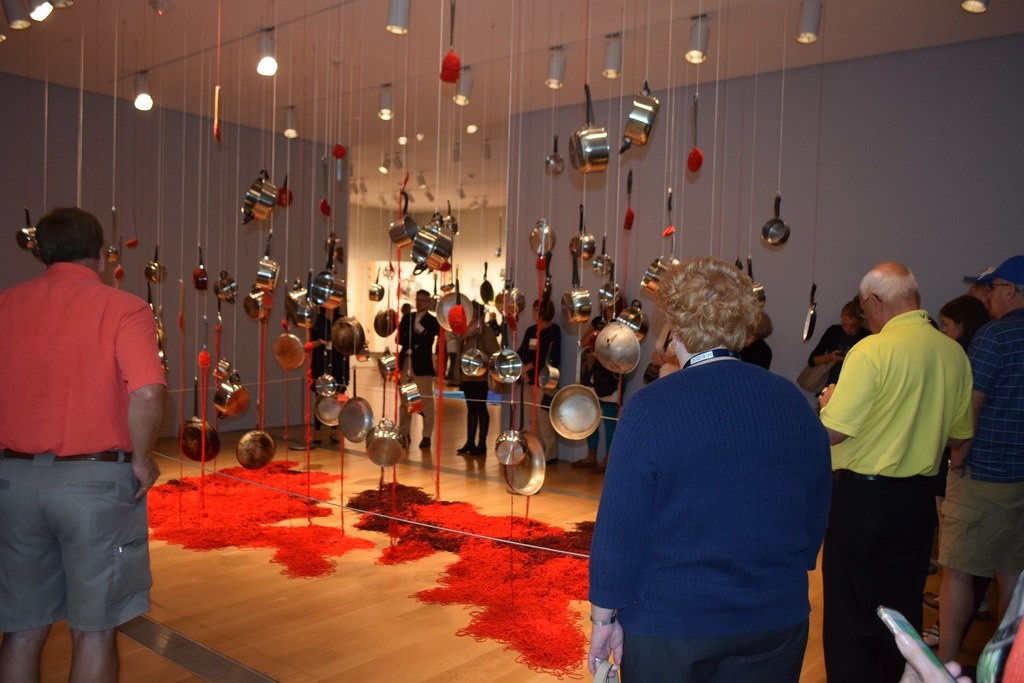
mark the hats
[964,266,995,285]
[980,255,1024,285]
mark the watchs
[948,457,965,469]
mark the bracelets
[590,609,617,625]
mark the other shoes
[572,459,598,468]
[457,442,475,452]
[977,600,994,620]
[590,465,606,473]
[923,620,941,647]
[470,444,486,455]
[924,592,942,608]
[419,437,430,447]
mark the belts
[835,469,887,481]
[3,449,132,462]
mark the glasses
[856,294,882,319]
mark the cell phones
[877,605,958,683]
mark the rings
[822,387,829,394]
[596,657,603,661]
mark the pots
[16,80,818,497]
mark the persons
[448,300,499,453]
[588,257,834,683]
[311,306,349,444]
[808,295,874,396]
[922,295,990,649]
[517,299,562,465]
[571,316,628,474]
[937,254,1024,664]
[743,311,772,369]
[394,290,438,448]
[0,208,168,683]
[818,262,974,683]
[895,569,1024,683]
[488,313,500,335]
[653,322,681,377]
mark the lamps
[546,50,566,89]
[685,18,710,64]
[602,40,623,78]
[453,68,474,105]
[379,86,394,119]
[132,74,154,111]
[961,0,989,13]
[350,142,494,204]
[256,32,279,76]
[794,0,822,43]
[385,0,409,34]
[0,0,75,42]
[283,107,298,138]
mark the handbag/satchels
[797,360,837,392]
[644,362,660,384]
